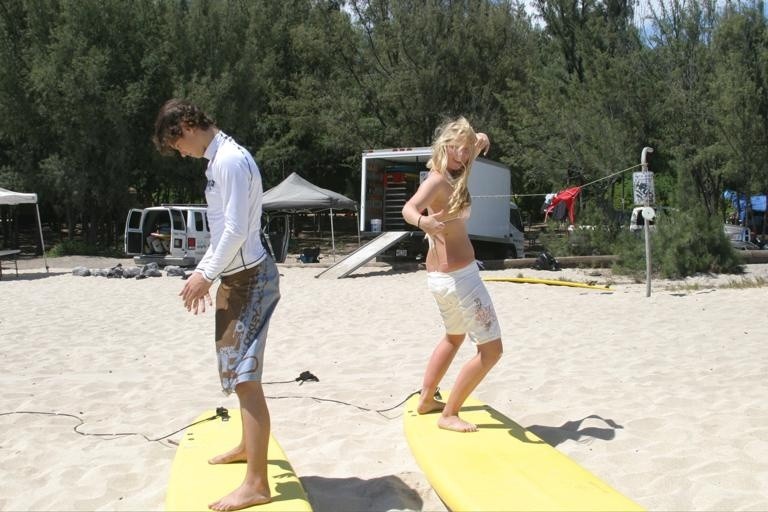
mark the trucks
[359,145,525,268]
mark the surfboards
[163,408,311,512]
[405,389,646,512]
[483,277,613,291]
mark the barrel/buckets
[370,218,383,232]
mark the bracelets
[417,215,424,231]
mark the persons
[151,97,280,512]
[400,116,504,434]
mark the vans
[123,202,291,265]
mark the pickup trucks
[565,205,752,249]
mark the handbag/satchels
[538,252,558,270]
